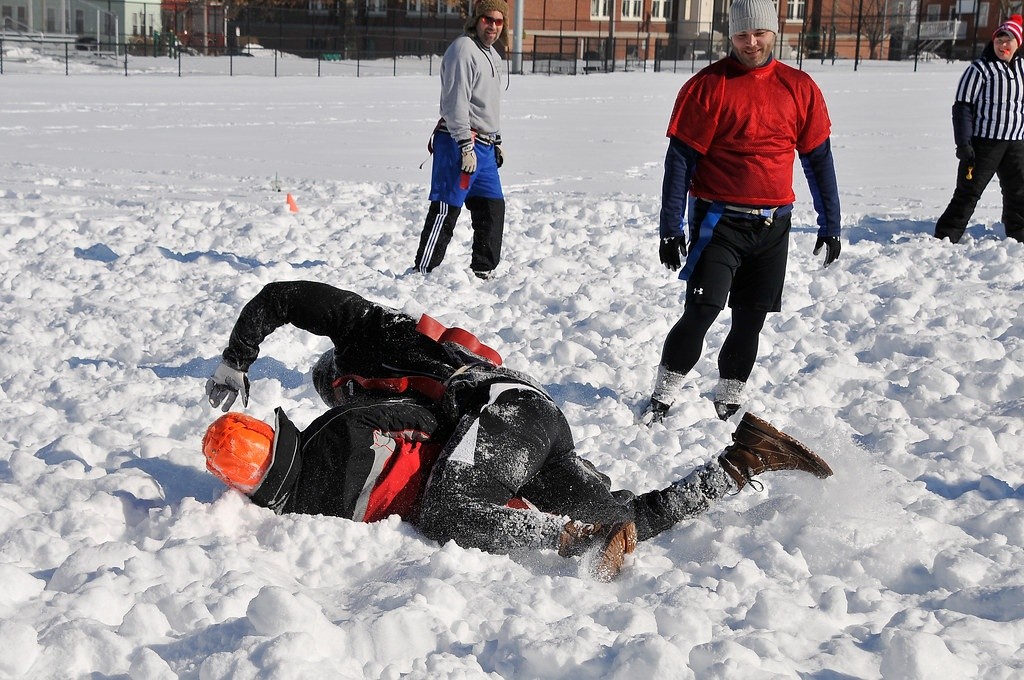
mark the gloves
[206,361,249,412]
[814,224,840,268]
[956,141,974,162]
[461,145,477,173]
[658,233,687,271]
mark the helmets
[313,342,340,409]
[202,413,275,495]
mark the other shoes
[641,399,671,428]
[714,402,740,421]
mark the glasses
[482,14,504,26]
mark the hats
[992,14,1024,49]
[464,0,509,91]
[728,0,779,40]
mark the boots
[557,521,638,585]
[717,412,834,489]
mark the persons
[643,0,841,430]
[202,394,638,522]
[207,280,831,582]
[412,0,510,282]
[935,14,1024,245]
